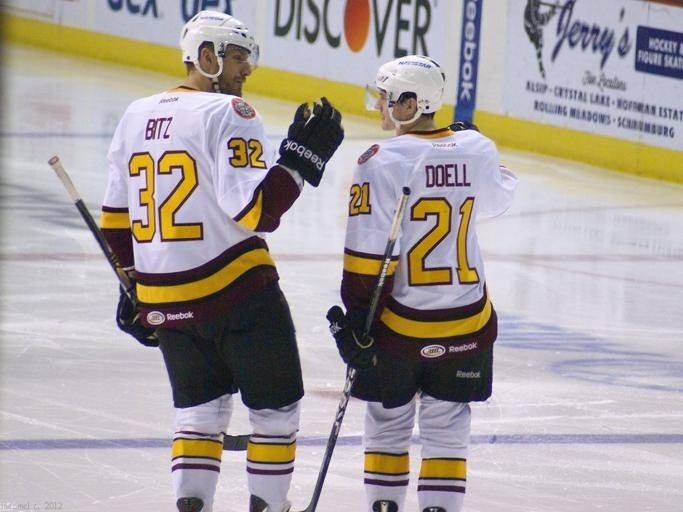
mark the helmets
[178,8,261,80]
[373,54,445,125]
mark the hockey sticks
[49,155,254,451]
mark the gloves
[324,302,377,372]
[449,120,481,133]
[116,277,161,347]
[275,94,347,188]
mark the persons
[340,54,518,512]
[101,10,344,512]
